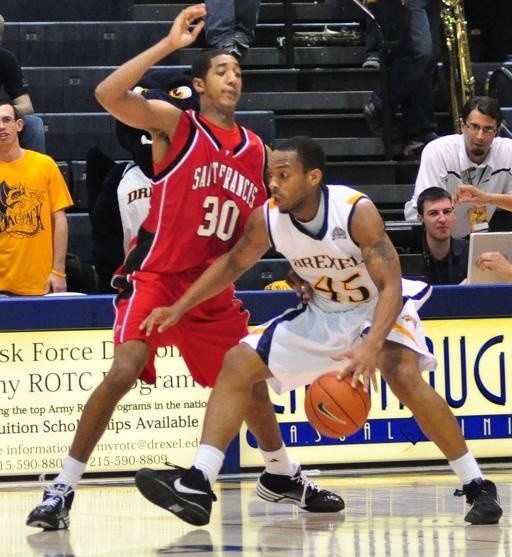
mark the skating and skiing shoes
[361,52,382,70]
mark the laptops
[466,228,512,286]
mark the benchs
[383,0,511,292]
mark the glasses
[464,121,498,134]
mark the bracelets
[51,268,67,278]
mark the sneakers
[232,27,257,49]
[25,529,76,557]
[214,37,243,60]
[152,529,214,557]
[253,462,348,515]
[360,95,404,139]
[133,461,219,527]
[25,481,75,533]
[452,475,505,525]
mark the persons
[452,182,512,282]
[1,14,45,156]
[24,2,346,532]
[360,0,443,160]
[199,0,260,58]
[403,93,512,240]
[133,135,503,527]
[414,182,468,285]
[0,97,75,297]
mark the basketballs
[304,372,372,440]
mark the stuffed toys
[88,67,200,293]
